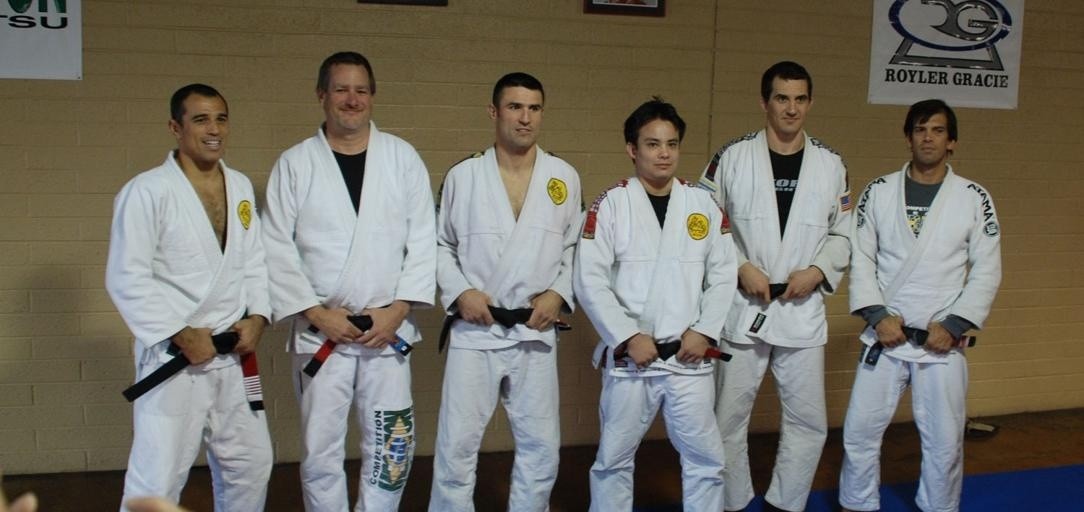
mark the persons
[838,98,1004,512]
[572,94,738,512]
[427,70,582,512]
[106,83,276,512]
[696,61,853,512]
[262,49,438,511]
